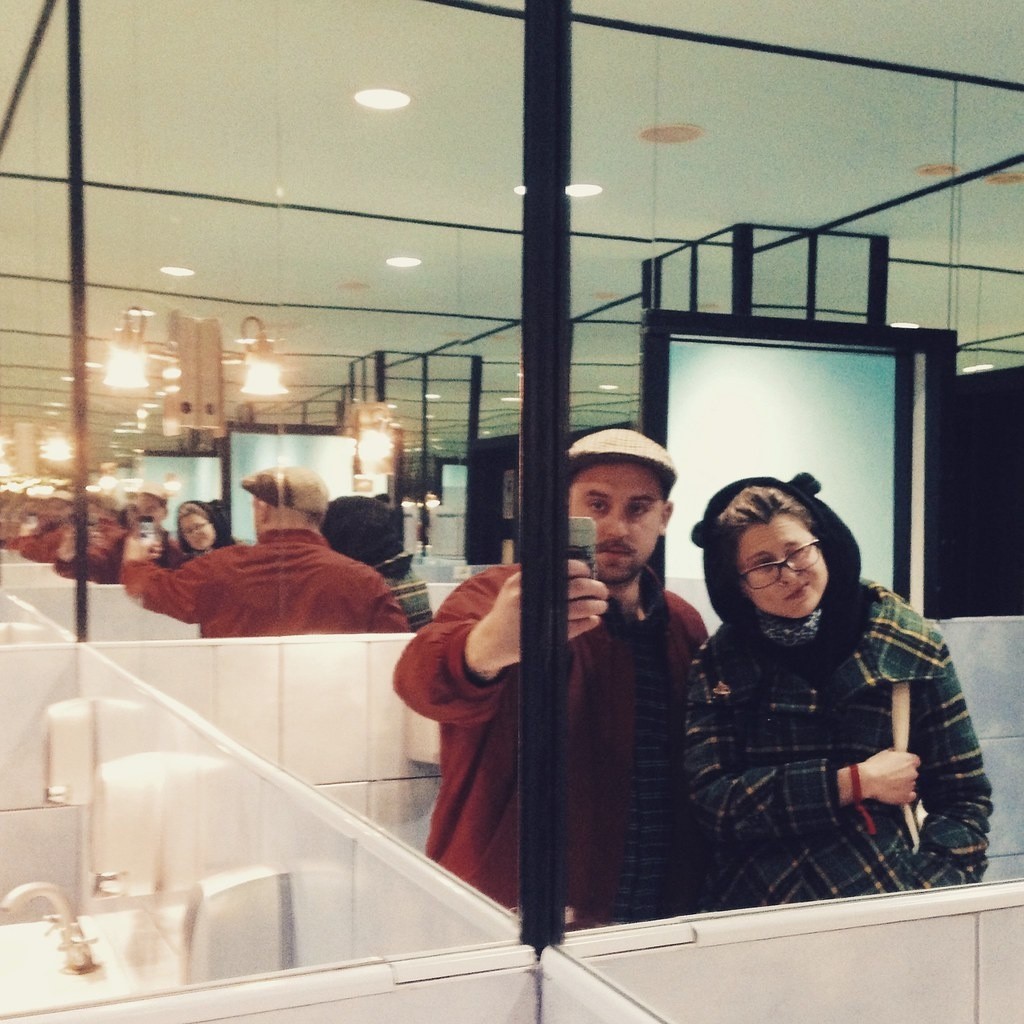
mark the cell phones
[136,516,158,543]
[566,515,598,584]
[26,513,39,525]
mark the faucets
[1,881,102,977]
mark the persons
[1,490,126,563]
[678,474,993,907]
[322,494,433,631]
[55,482,181,583]
[177,500,242,563]
[392,427,710,931]
[123,467,409,638]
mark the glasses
[725,538,821,589]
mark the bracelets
[849,764,876,835]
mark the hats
[137,483,169,502]
[241,467,329,517]
[568,428,676,502]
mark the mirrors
[541,12,1024,953]
[0,5,521,909]
